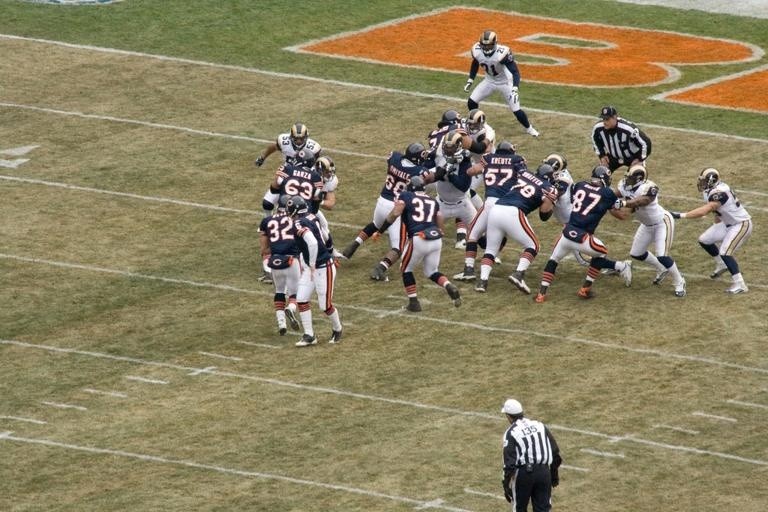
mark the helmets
[406,176,425,191]
[623,166,647,191]
[439,110,486,163]
[591,166,612,188]
[697,168,720,192]
[277,195,308,218]
[537,154,567,183]
[405,143,426,165]
[290,123,336,183]
[479,32,497,56]
[496,141,516,152]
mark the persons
[669,168,754,293]
[255,122,344,346]
[463,29,541,137]
[343,108,686,313]
[500,399,563,512]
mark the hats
[599,107,616,119]
[502,399,522,415]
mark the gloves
[255,157,264,166]
[503,480,512,502]
[463,83,471,93]
[550,465,559,487]
[508,87,519,103]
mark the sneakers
[526,125,539,137]
[710,261,728,278]
[508,270,531,294]
[535,292,547,302]
[578,287,595,298]
[724,285,748,295]
[453,238,501,293]
[256,272,273,283]
[370,266,385,280]
[445,281,461,307]
[653,266,686,297]
[278,306,343,346]
[618,260,632,286]
[407,300,420,312]
[343,247,353,257]
